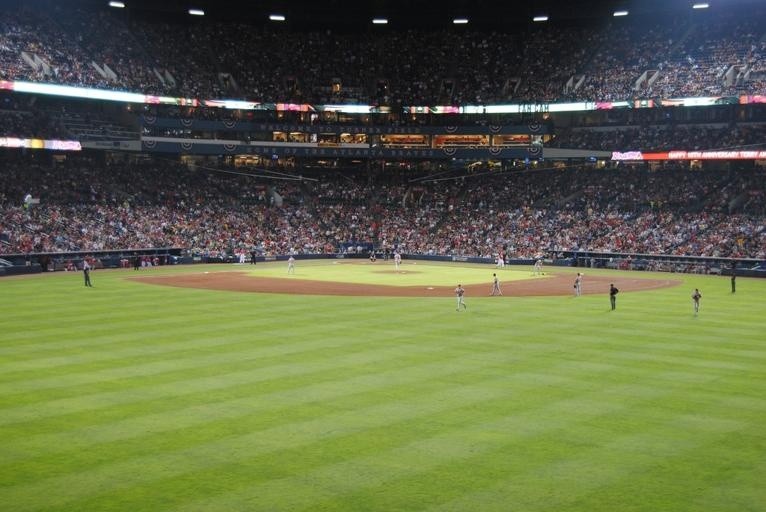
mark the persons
[574,273,585,297]
[610,283,620,310]
[691,287,703,314]
[287,255,296,275]
[489,272,503,296]
[731,272,737,293]
[454,283,467,312]
[2,2,765,272]
[82,259,93,287]
[531,257,544,276]
